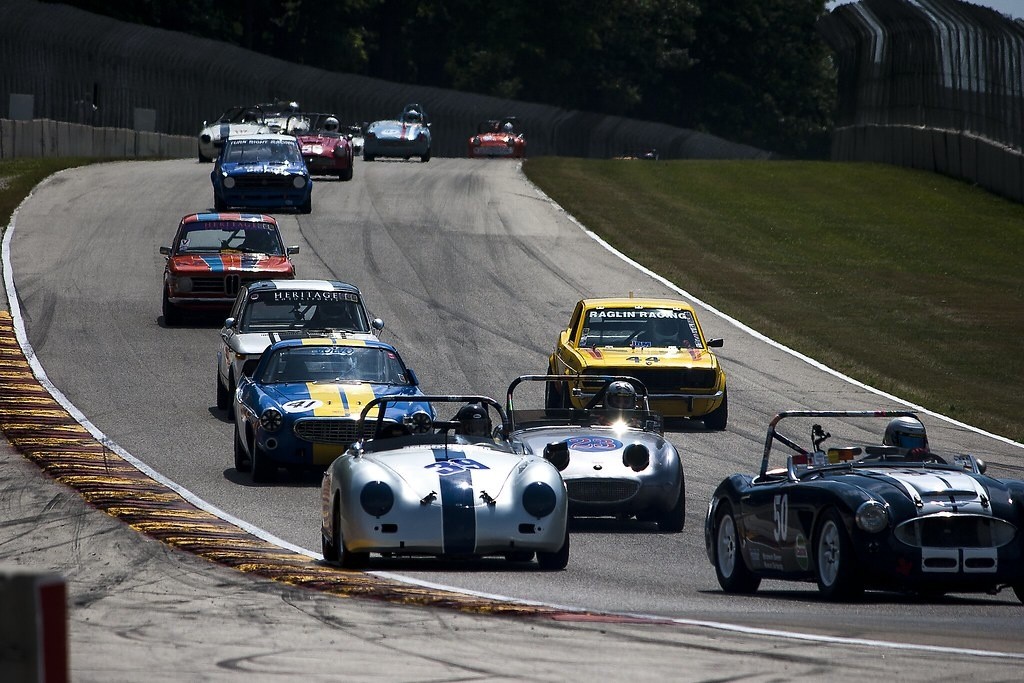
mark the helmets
[654,315,680,341]
[457,404,490,439]
[407,110,422,124]
[505,122,513,133]
[323,117,339,133]
[606,381,636,414]
[885,416,928,454]
[318,301,348,321]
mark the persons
[591,381,643,427]
[353,355,386,382]
[404,110,421,123]
[318,302,344,320]
[503,122,514,133]
[324,116,340,133]
[650,318,692,348]
[239,229,277,251]
[272,144,292,160]
[286,102,299,116]
[243,111,258,124]
[456,404,494,438]
[881,417,930,461]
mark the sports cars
[703,408,1024,606]
[544,296,729,432]
[362,103,433,162]
[254,100,310,135]
[197,106,282,163]
[232,337,438,482]
[210,133,313,213]
[467,116,528,158]
[215,279,385,420]
[159,211,300,328]
[494,373,687,532]
[280,111,354,181]
[319,393,572,572]
